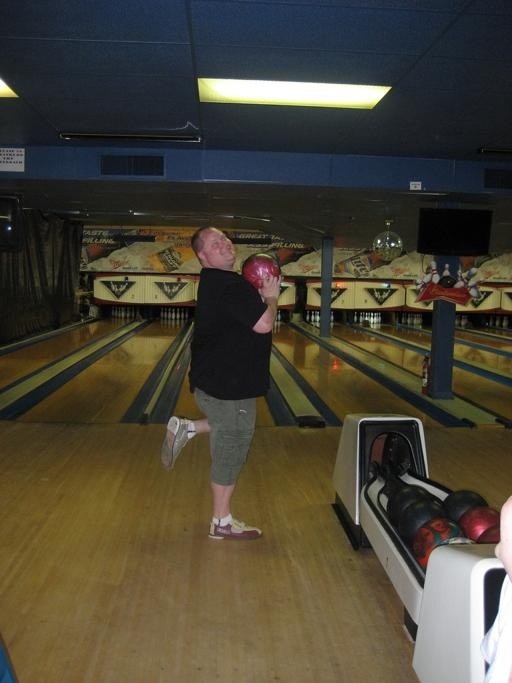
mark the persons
[163,227,283,541]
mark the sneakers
[160,416,189,470]
[208,518,262,539]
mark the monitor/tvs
[416,206,493,256]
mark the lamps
[371,217,403,261]
